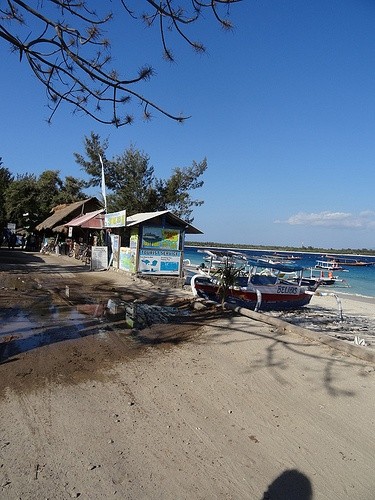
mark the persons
[2,229,41,252]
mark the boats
[182,242,375,311]
